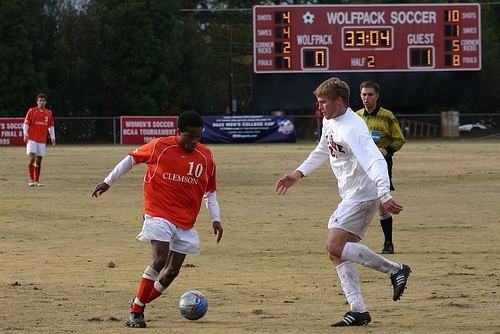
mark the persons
[315,100,324,143]
[355,81,405,254]
[23,93,55,186]
[275,77,412,326]
[91,111,224,327]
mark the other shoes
[28,180,43,187]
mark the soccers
[179,290,208,321]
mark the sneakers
[124,312,146,328]
[381,241,394,254]
[330,310,371,327]
[128,296,146,319]
[389,264,412,302]
